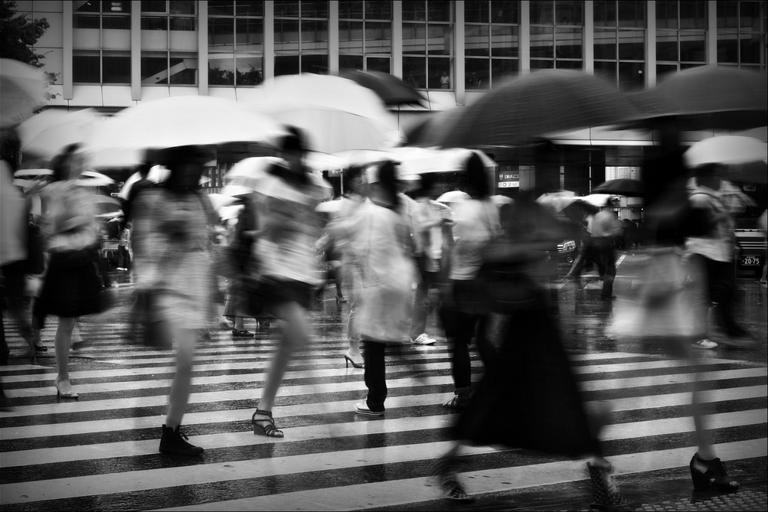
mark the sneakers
[354,402,385,416]
[693,339,719,348]
[410,333,436,344]
[232,329,254,337]
[159,424,204,456]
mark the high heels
[691,453,740,501]
[252,408,284,438]
[344,352,366,368]
[55,378,79,399]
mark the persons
[0,121,766,511]
